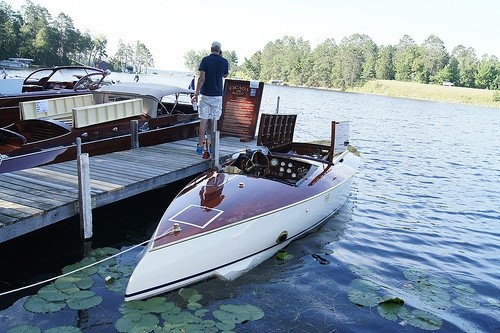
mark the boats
[123,120,365,303]
[0,82,285,243]
[0,57,35,71]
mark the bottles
[192,98,198,111]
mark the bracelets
[194,92,199,96]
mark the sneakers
[196,144,203,155]
[210,145,212,154]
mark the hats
[211,41,221,48]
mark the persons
[191,40,228,154]
